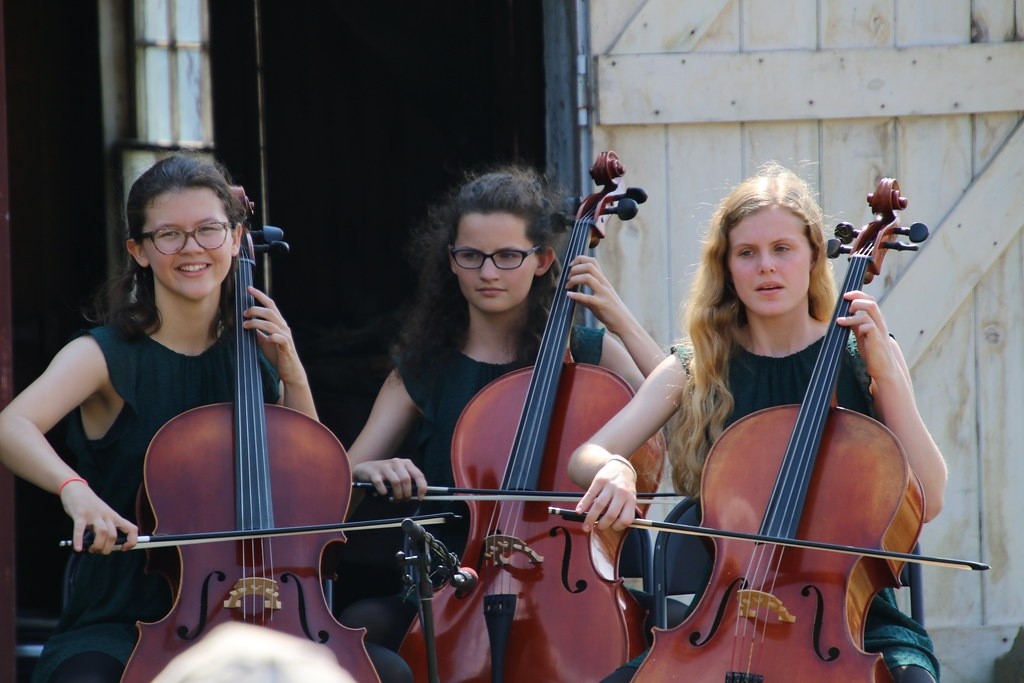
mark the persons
[345,172,672,592]
[565,173,949,682]
[1,157,320,682]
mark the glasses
[138,221,232,255]
[451,244,541,270]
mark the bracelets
[56,477,88,497]
[606,454,638,482]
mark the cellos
[397,152,667,683]
[629,177,926,683]
[116,185,382,683]
